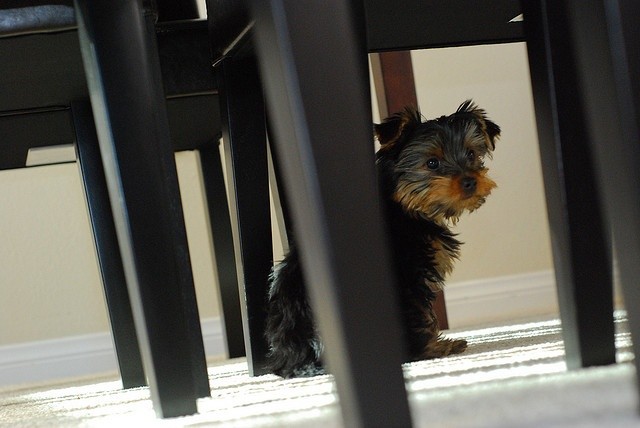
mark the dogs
[261,98,501,380]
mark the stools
[74,0,616,427]
[0,0,277,392]
[69,18,219,390]
